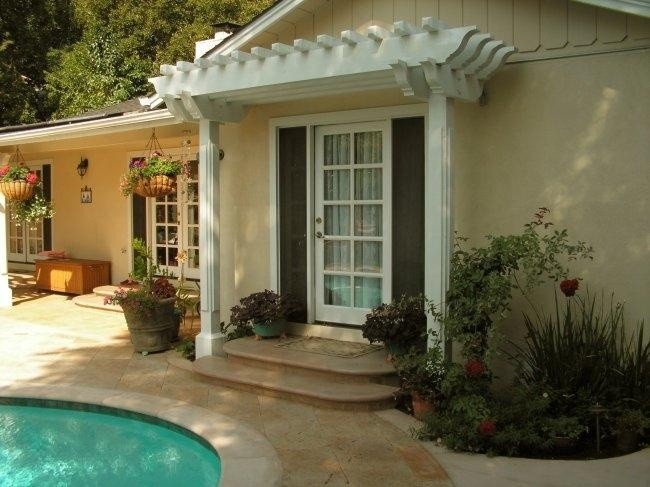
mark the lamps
[77,160,87,177]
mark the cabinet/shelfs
[35,257,111,296]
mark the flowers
[0,150,55,228]
[121,133,191,197]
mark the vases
[1,180,35,201]
[132,174,176,197]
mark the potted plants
[394,347,447,419]
[230,291,298,338]
[105,239,177,353]
[362,304,428,357]
